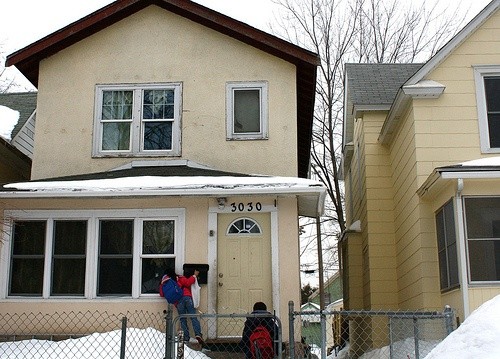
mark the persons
[237,302,286,359]
[159,270,210,349]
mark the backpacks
[162,274,183,304]
[249,327,274,359]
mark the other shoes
[196,335,206,347]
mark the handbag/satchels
[191,275,201,309]
